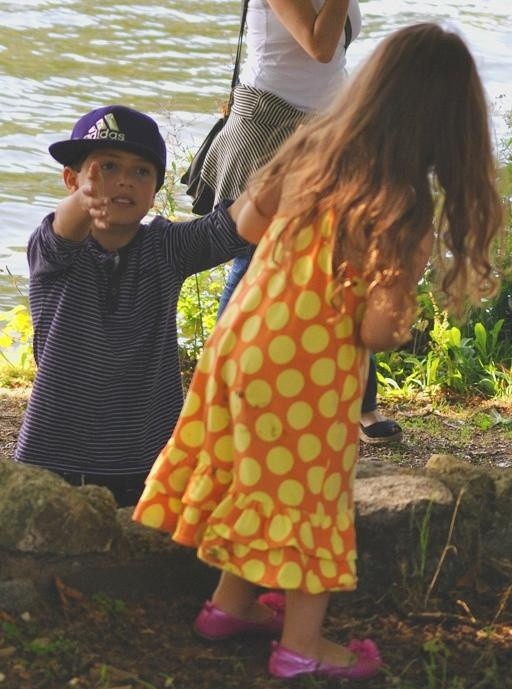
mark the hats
[47,104,167,192]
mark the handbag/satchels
[180,1,352,214]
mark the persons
[197,1,404,449]
[129,22,508,682]
[12,104,306,511]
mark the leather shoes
[193,592,285,640]
[267,637,382,680]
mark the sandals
[359,417,405,445]
